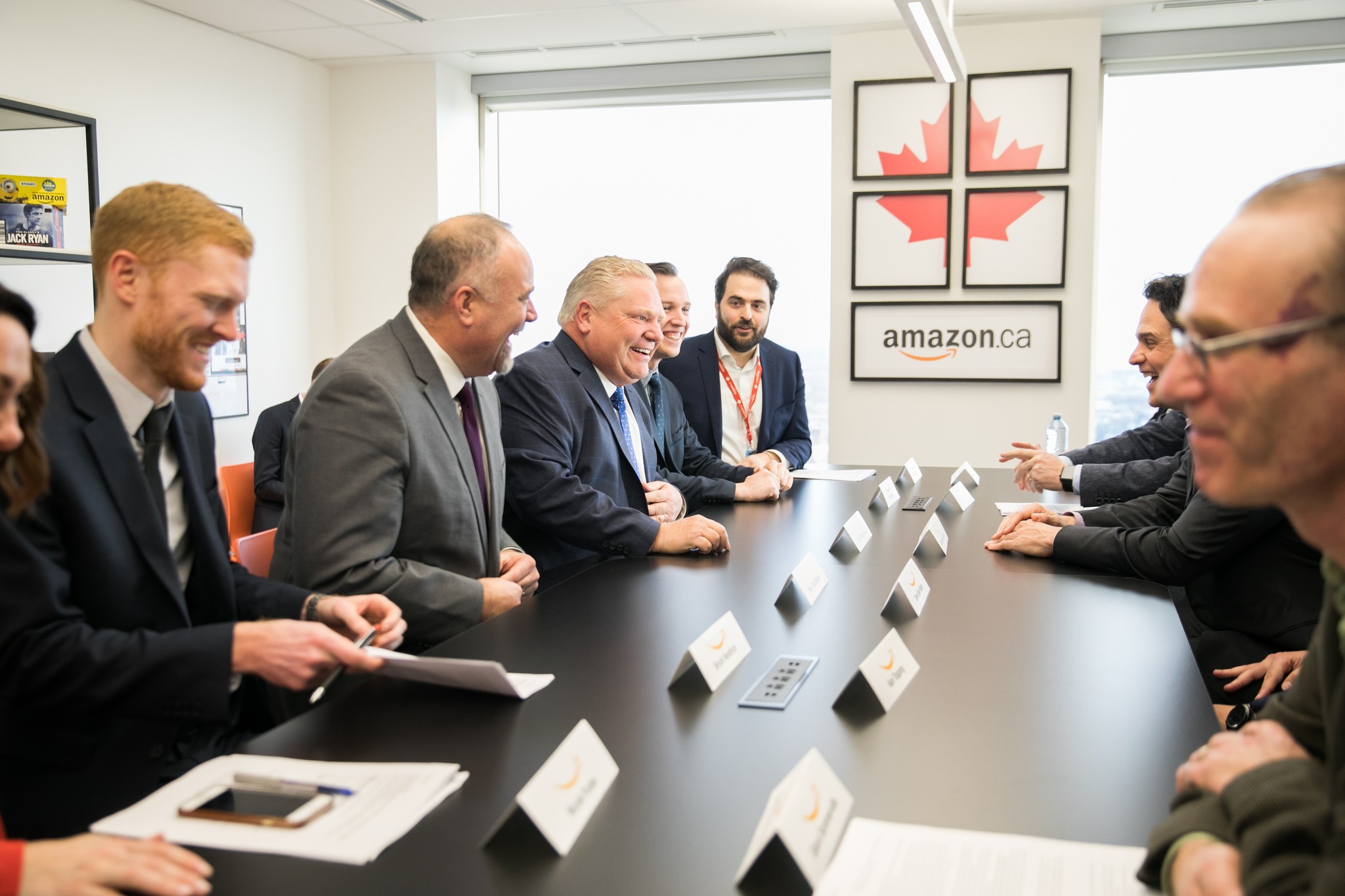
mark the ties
[611,385,640,487]
[456,380,488,511]
[648,372,664,456]
[140,400,174,561]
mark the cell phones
[179,782,335,828]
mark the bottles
[1046,414,1067,456]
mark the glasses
[1171,311,1342,378]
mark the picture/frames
[852,78,955,182]
[849,299,1062,385]
[961,186,1069,288]
[964,66,1071,176]
[850,188,952,290]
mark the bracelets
[304,594,330,622]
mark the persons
[494,253,731,598]
[249,357,335,536]
[997,273,1193,507]
[0,181,407,838]
[1189,626,1315,733]
[0,282,216,896]
[266,212,544,654]
[639,262,780,510]
[658,256,811,474]
[984,416,1325,709]
[1133,162,1345,896]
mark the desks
[110,464,1223,895]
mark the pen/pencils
[234,772,354,798]
[307,622,382,705]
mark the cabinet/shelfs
[0,94,103,363]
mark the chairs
[235,526,278,579]
[220,462,256,550]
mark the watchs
[1224,703,1256,733]
[1059,465,1075,493]
